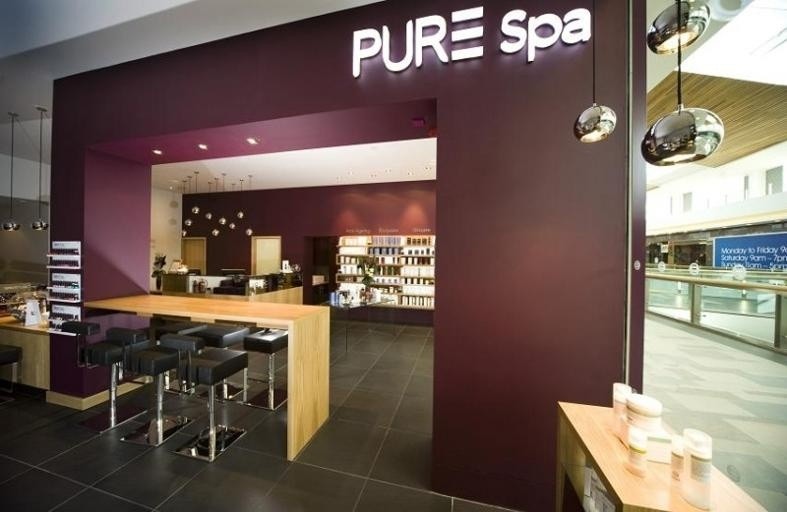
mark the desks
[554,399,767,512]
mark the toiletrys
[582,379,713,512]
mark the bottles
[609,381,666,478]
[668,436,684,484]
[681,428,712,510]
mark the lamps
[572,2,724,165]
[1,104,49,233]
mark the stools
[0,344,21,400]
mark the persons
[35,281,48,313]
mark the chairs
[60,315,287,463]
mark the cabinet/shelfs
[335,234,436,311]
